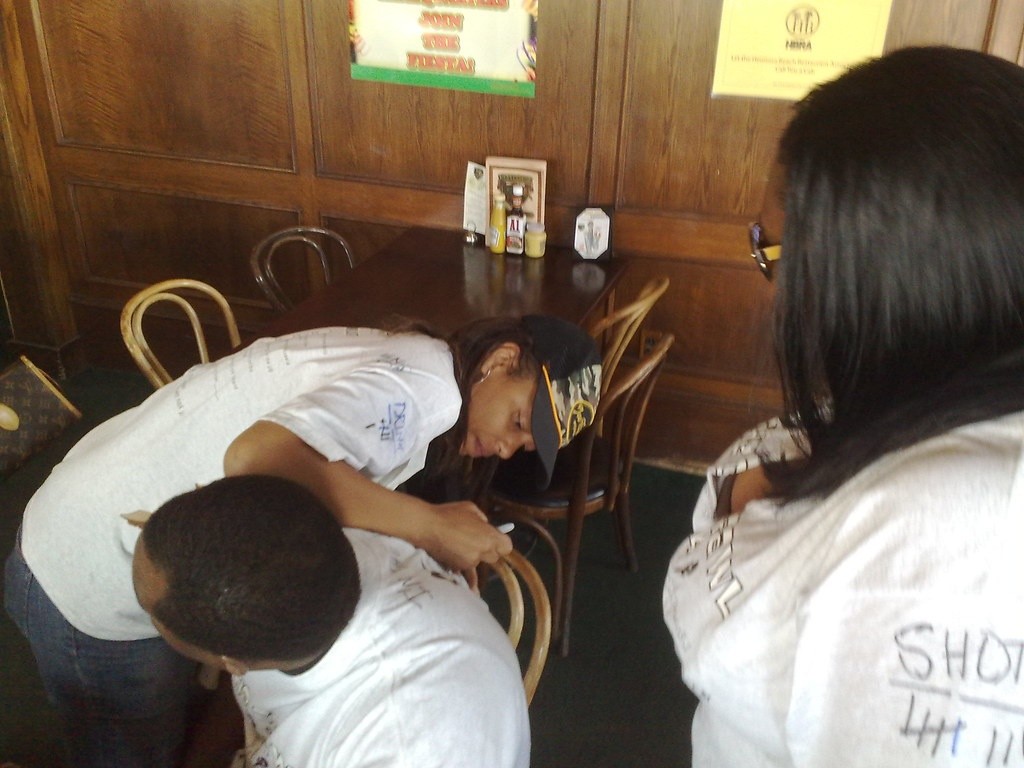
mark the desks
[233,224,630,351]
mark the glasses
[748,222,783,283]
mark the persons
[3,311,603,768]
[661,44,1024,768]
[130,472,533,768]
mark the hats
[522,314,602,491]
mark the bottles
[488,185,548,259]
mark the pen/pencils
[495,522,515,534]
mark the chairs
[486,273,679,710]
[252,225,357,317]
[119,277,242,392]
